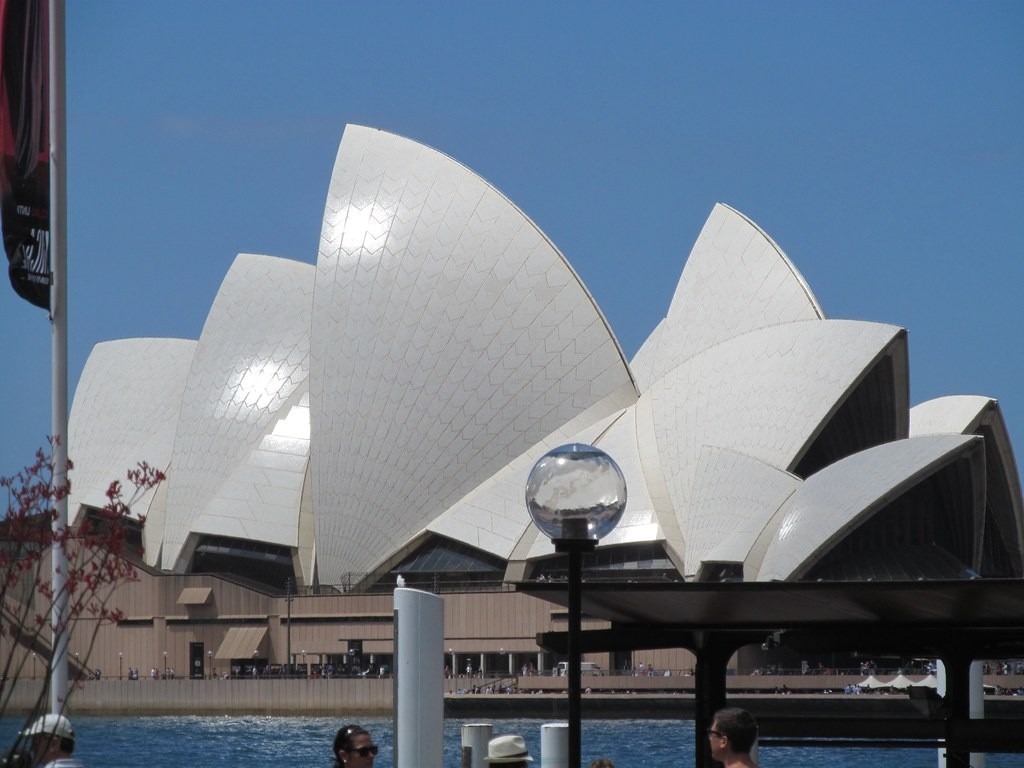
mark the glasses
[350,746,378,756]
[706,728,722,738]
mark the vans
[556,660,601,676]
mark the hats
[18,714,76,740]
[483,735,534,763]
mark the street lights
[524,441,629,768]
[499,647,507,679]
[283,578,293,679]
[32,652,36,680]
[301,649,306,679]
[207,650,213,680]
[448,648,455,677]
[254,650,259,669]
[118,652,122,680]
[75,652,79,674]
[349,649,355,676]
[163,650,167,679]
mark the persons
[709,707,762,768]
[536,573,569,583]
[332,724,379,768]
[96,662,396,681]
[16,712,86,768]
[442,658,1024,701]
[482,735,534,768]
